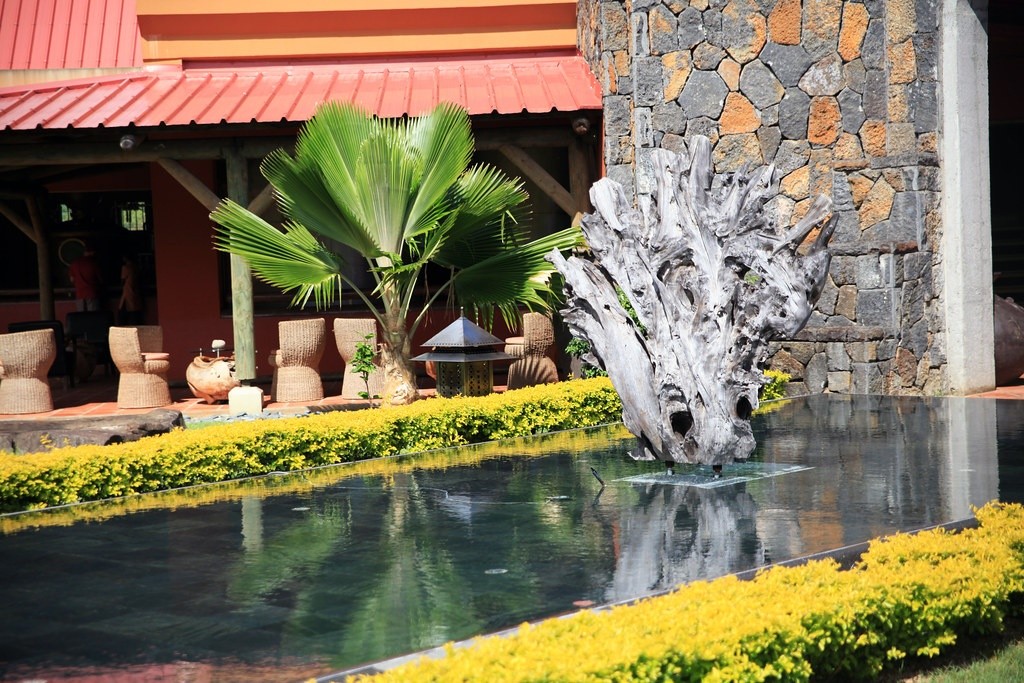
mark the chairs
[505,309,558,391]
[267,318,325,402]
[0,328,58,415]
[108,326,172,408]
[6,307,119,391]
[333,318,383,400]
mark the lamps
[406,305,523,398]
[120,135,134,148]
[572,116,587,132]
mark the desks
[184,348,240,403]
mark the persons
[67,243,150,325]
